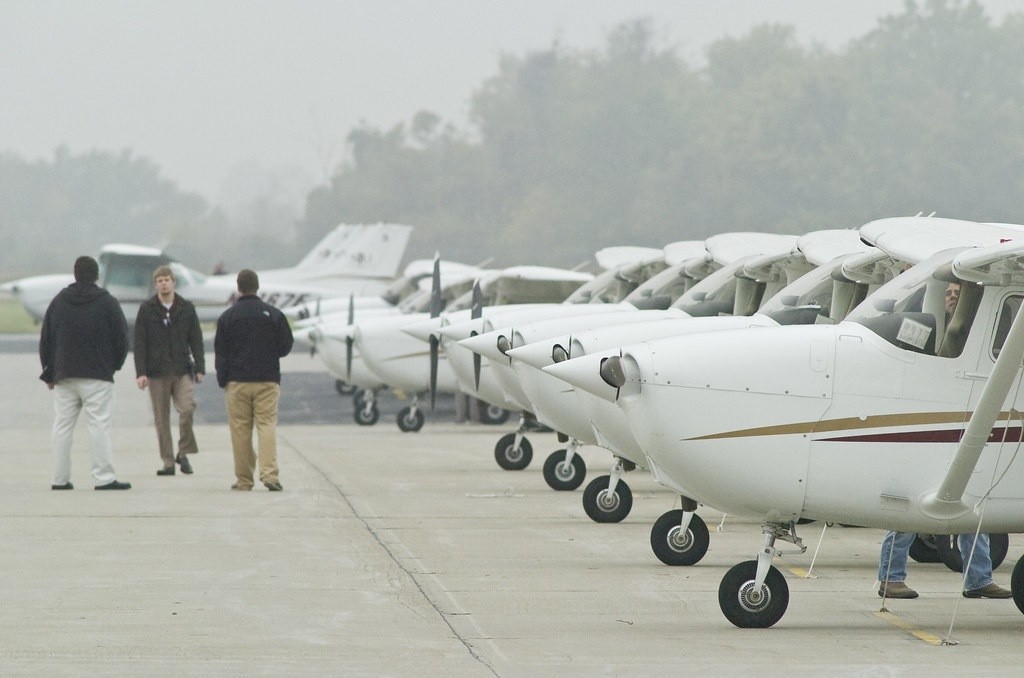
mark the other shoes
[264,480,283,490]
[176,452,194,474]
[962,583,1013,599]
[878,581,919,599]
[94,479,131,490]
[232,484,239,489]
[157,466,176,475]
[51,482,73,490]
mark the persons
[134,265,205,476]
[214,270,294,491]
[877,282,1013,599]
[38,256,132,490]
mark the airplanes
[0,212,1024,628]
